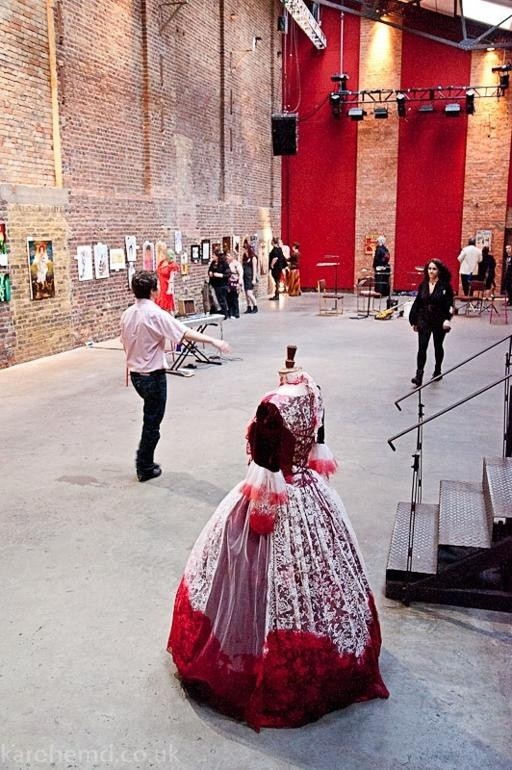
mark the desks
[164,314,226,378]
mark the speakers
[374,266,390,296]
[387,300,398,310]
[272,112,299,156]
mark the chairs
[317,276,383,317]
[451,280,486,318]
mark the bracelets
[169,280,175,284]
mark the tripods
[455,286,500,324]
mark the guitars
[375,298,411,320]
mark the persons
[153,247,178,316]
[409,257,456,387]
[120,269,232,483]
[371,235,392,297]
[168,345,389,738]
[457,238,483,296]
[206,235,300,319]
[477,246,495,296]
[36,245,49,289]
[500,243,512,307]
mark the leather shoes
[154,463,159,468]
[272,294,279,299]
[217,310,239,320]
[139,469,161,482]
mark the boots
[432,366,442,381]
[412,370,423,385]
[245,306,251,313]
[251,306,257,313]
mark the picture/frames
[190,238,212,266]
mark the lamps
[328,63,511,120]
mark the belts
[422,304,435,312]
